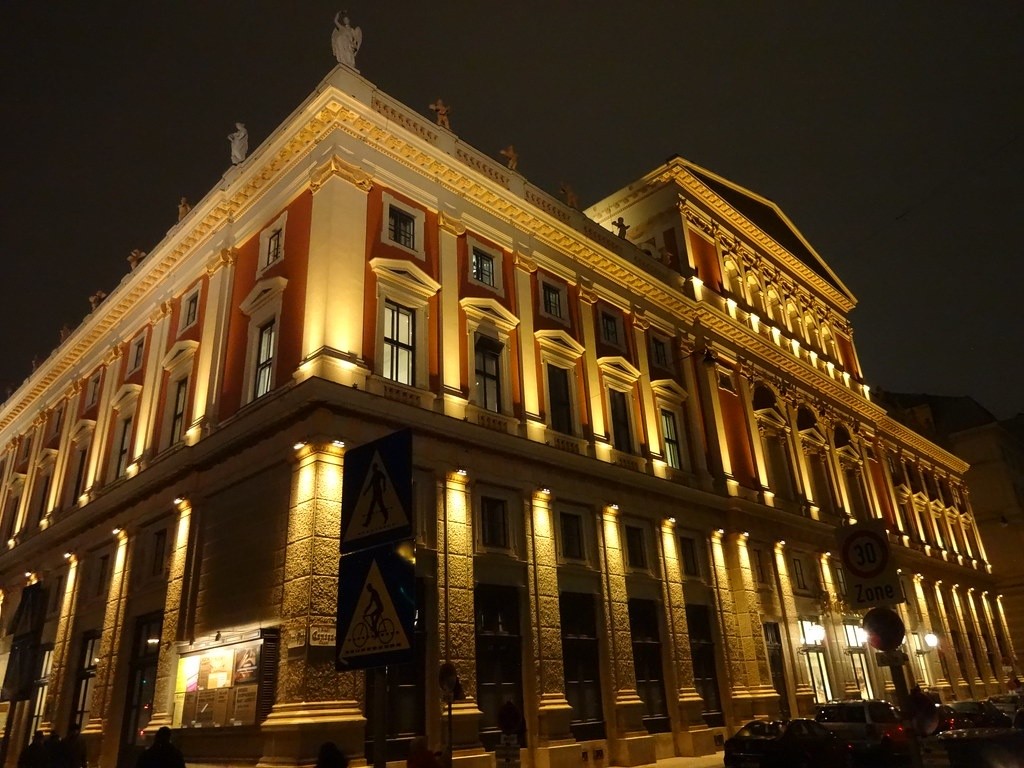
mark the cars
[928,690,1024,763]
[722,717,860,768]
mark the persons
[139,726,186,768]
[178,198,191,220]
[18,725,88,768]
[89,290,106,311]
[331,11,363,67]
[317,743,349,768]
[429,99,451,129]
[128,250,146,270]
[499,145,518,170]
[613,217,630,240]
[228,122,249,164]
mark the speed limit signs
[834,518,895,580]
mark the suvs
[814,698,910,765]
